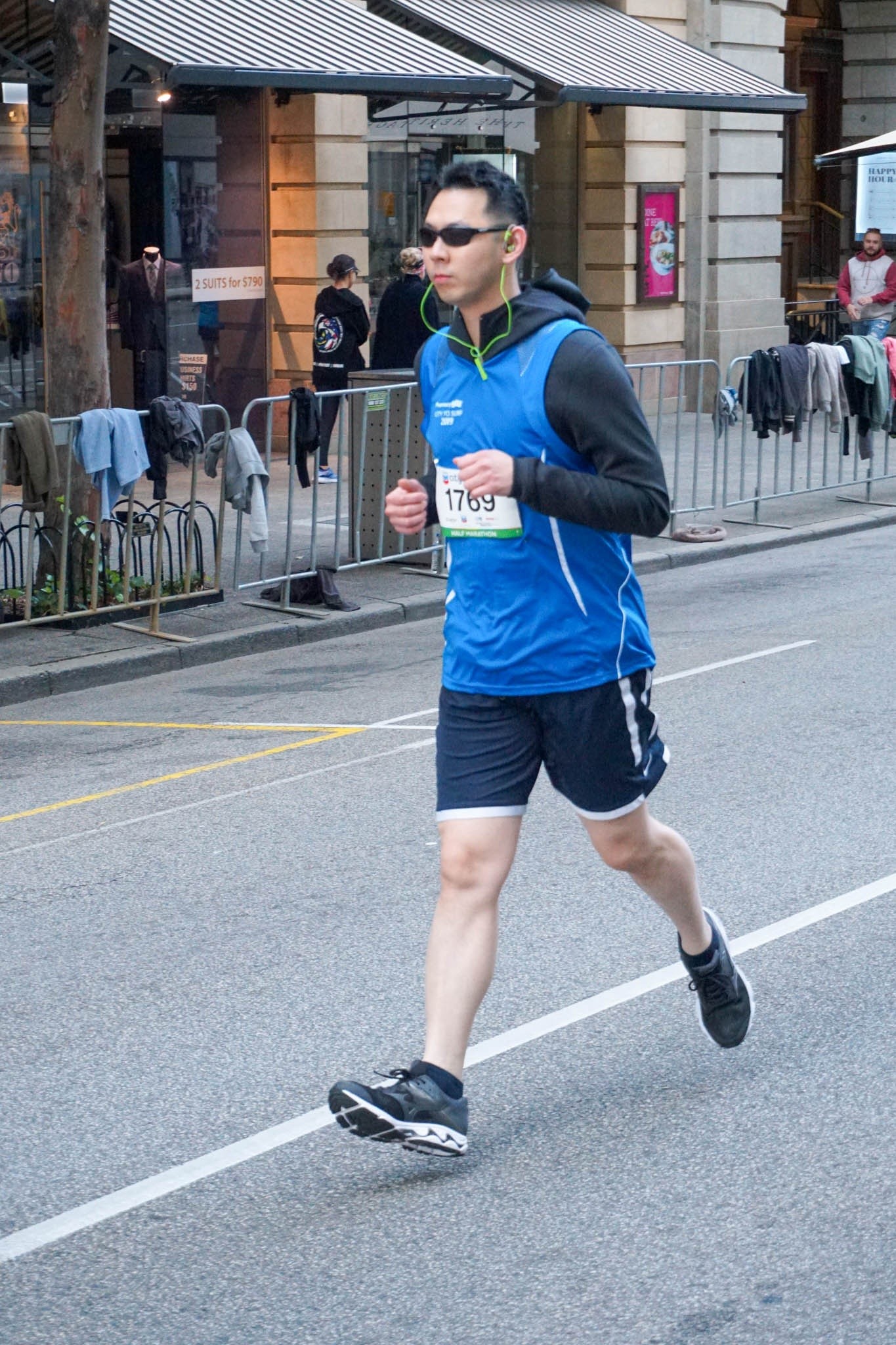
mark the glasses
[418,224,508,247]
[867,228,880,233]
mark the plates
[651,220,675,242]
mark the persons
[118,246,189,409]
[194,246,222,399]
[375,246,442,371]
[310,253,372,483]
[325,161,753,1160]
[837,228,896,340]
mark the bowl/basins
[649,242,675,275]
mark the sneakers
[677,907,756,1049]
[311,467,338,484]
[327,1064,469,1155]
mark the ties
[147,263,156,298]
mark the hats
[339,262,361,274]
[407,246,425,270]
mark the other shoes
[205,384,215,403]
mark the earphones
[507,244,516,251]
